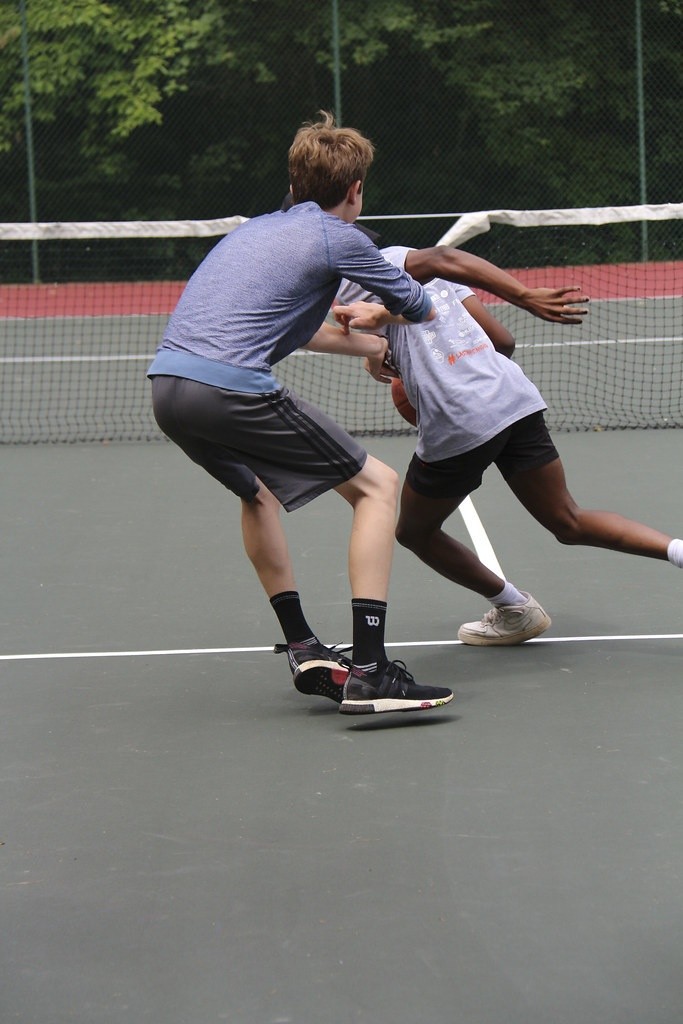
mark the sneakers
[273,641,352,704]
[458,591,552,645]
[337,658,453,715]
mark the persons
[146,106,455,715]
[278,186,683,648]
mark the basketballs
[391,376,417,427]
